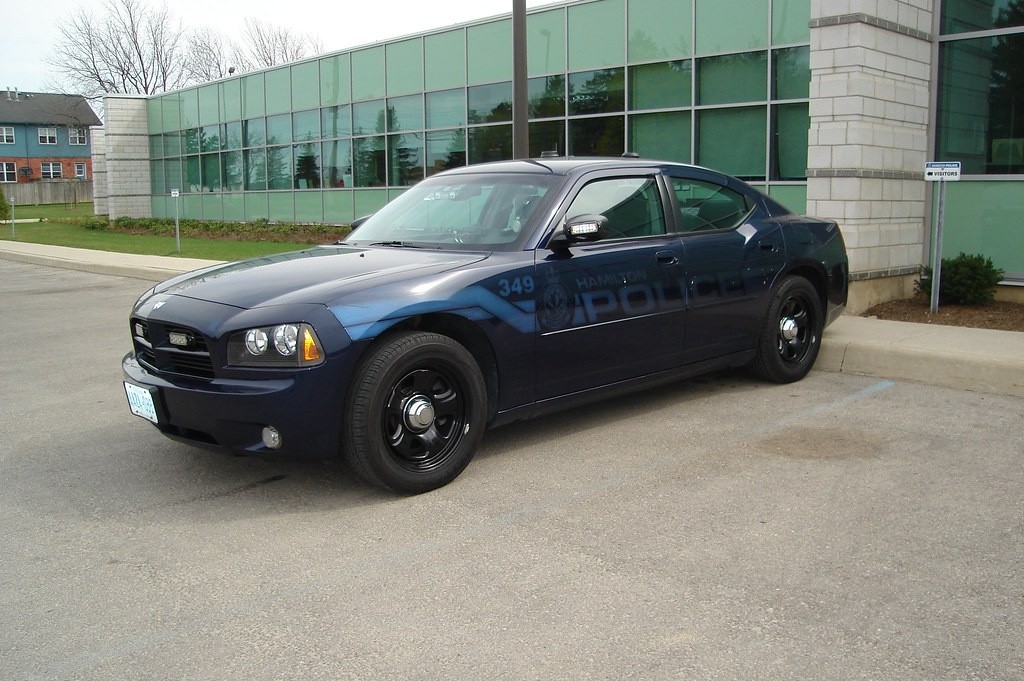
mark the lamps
[228,67,235,76]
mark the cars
[122,157,849,498]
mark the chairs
[507,196,543,238]
